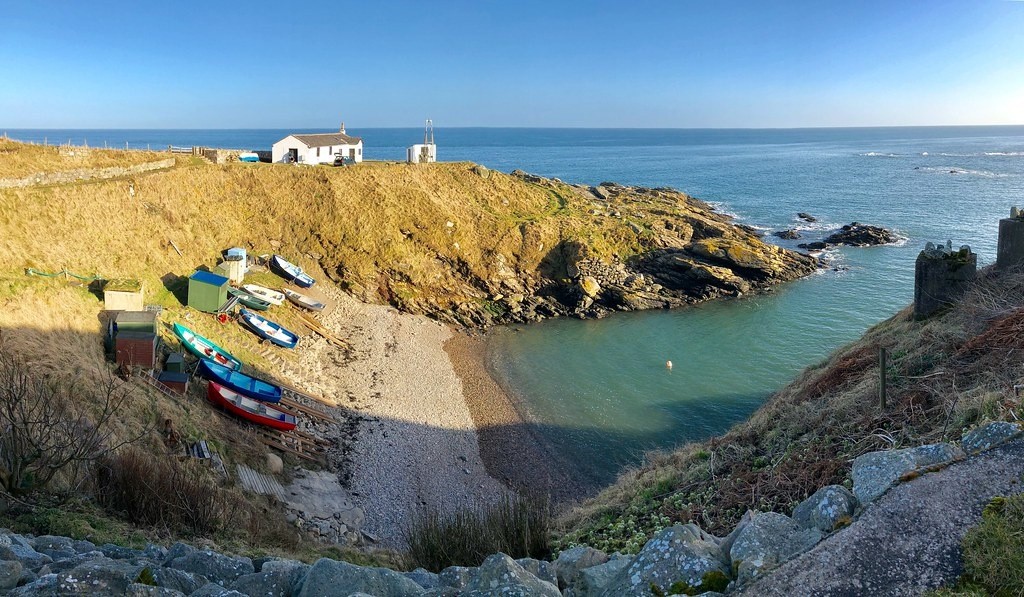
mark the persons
[161,417,181,446]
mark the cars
[334,153,357,166]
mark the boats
[174,322,242,373]
[206,380,297,431]
[243,285,285,305]
[226,284,270,311]
[239,308,299,349]
[199,356,283,404]
[273,254,317,287]
[280,286,327,313]
